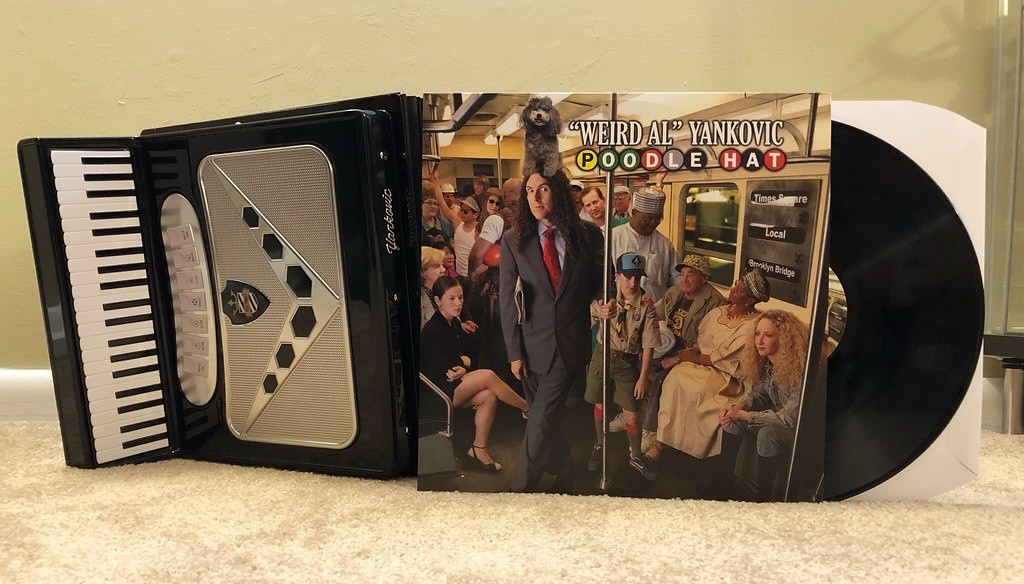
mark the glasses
[735,279,747,294]
[424,202,438,206]
[460,209,474,215]
[615,195,628,201]
[636,212,663,226]
[570,186,581,192]
[488,198,502,207]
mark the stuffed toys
[521,97,563,175]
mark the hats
[615,185,630,196]
[504,178,523,202]
[440,183,455,193]
[742,267,770,303]
[674,255,713,278]
[570,180,584,190]
[459,196,480,213]
[617,252,649,279]
[633,187,666,214]
[487,187,502,199]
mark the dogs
[517,96,563,177]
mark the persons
[498,169,617,493]
[570,177,822,503]
[421,175,530,474]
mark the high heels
[521,410,530,426]
[465,444,502,473]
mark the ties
[616,300,635,337]
[543,228,562,298]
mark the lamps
[484,93,573,146]
[437,105,455,147]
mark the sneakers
[590,413,664,481]
